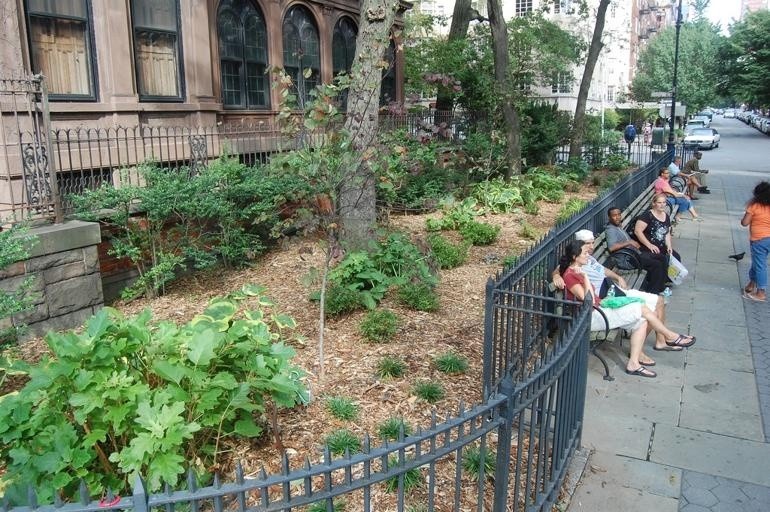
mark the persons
[561,240,657,378]
[655,168,699,219]
[552,229,697,351]
[684,152,710,194]
[643,123,653,146]
[604,207,672,297]
[741,182,770,301]
[668,156,707,200]
[634,194,682,288]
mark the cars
[682,107,770,150]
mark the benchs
[543,170,701,381]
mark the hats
[575,230,594,242]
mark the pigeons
[728,252,745,262]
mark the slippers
[628,351,656,365]
[626,367,657,377]
[743,285,766,301]
[654,335,696,351]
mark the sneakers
[697,186,711,194]
[693,215,704,220]
[675,217,682,224]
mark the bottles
[607,284,616,297]
